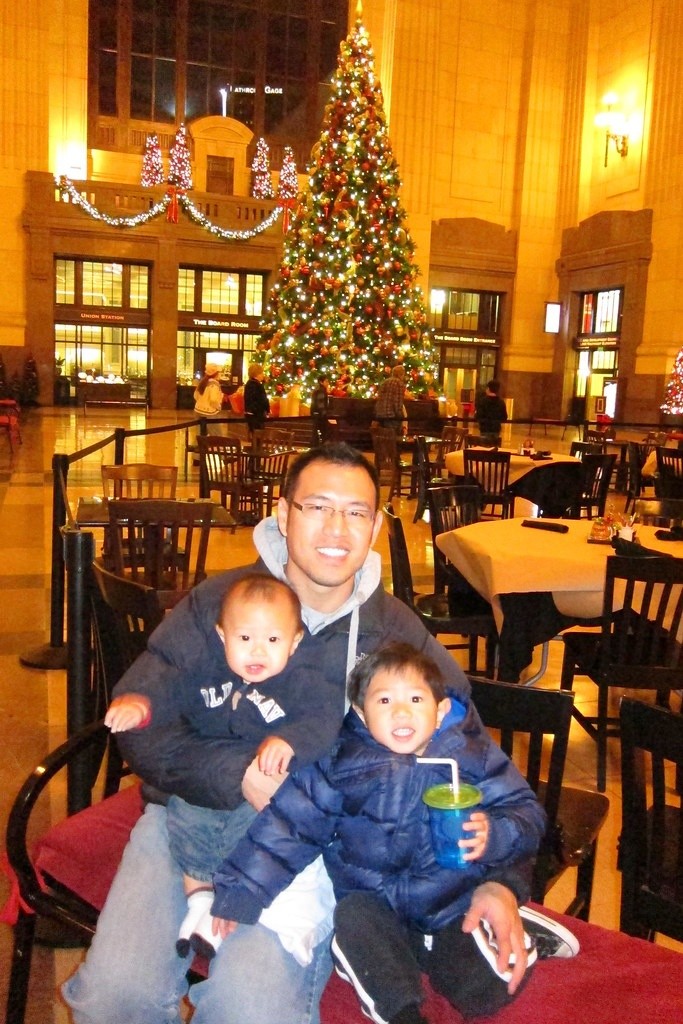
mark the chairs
[0,399,683,950]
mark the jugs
[590,519,612,540]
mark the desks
[435,517,683,758]
[594,438,660,495]
[196,445,297,526]
[443,447,583,491]
[378,433,453,501]
[641,450,681,479]
[76,496,237,583]
[113,568,229,611]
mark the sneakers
[517,906,580,960]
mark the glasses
[289,498,374,526]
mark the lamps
[596,89,627,168]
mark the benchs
[528,411,583,442]
[1,720,682,1024]
[82,390,151,419]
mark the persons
[244,365,270,470]
[104,575,344,958]
[211,644,581,1024]
[309,375,330,444]
[474,379,508,440]
[60,443,472,1024]
[194,364,227,465]
[372,365,407,468]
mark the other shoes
[246,463,263,470]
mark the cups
[422,781,483,870]
[618,527,633,542]
[621,513,633,527]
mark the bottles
[607,504,620,530]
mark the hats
[205,363,218,376]
[248,365,263,378]
